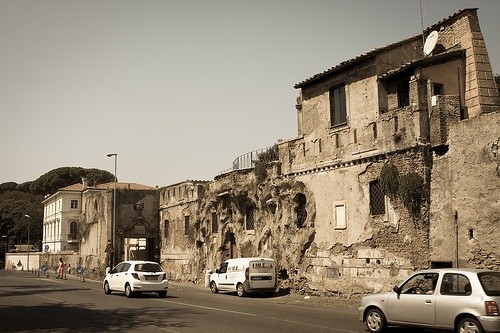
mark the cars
[102,260,169,298]
[357,267,500,333]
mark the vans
[209,257,278,297]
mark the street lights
[106,153,118,270]
[24,214,31,272]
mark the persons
[416,279,437,294]
[56,258,63,279]
